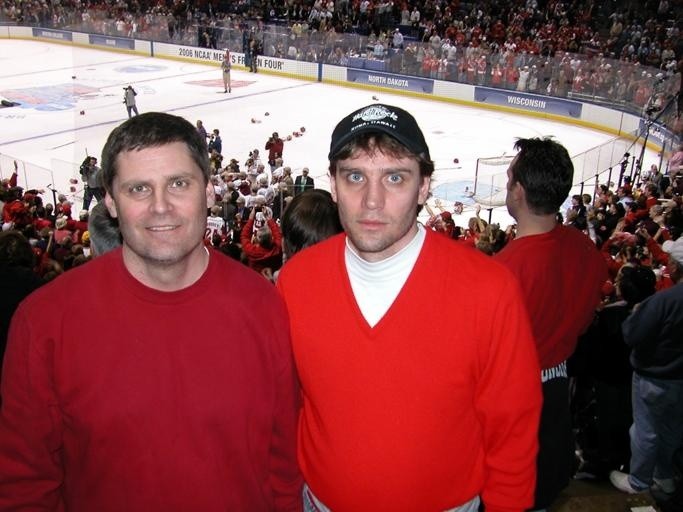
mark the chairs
[202,18,424,74]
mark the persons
[1,1,683,512]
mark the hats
[327,104,430,159]
[22,194,90,254]
[663,237,683,265]
[625,201,637,210]
[209,150,309,215]
